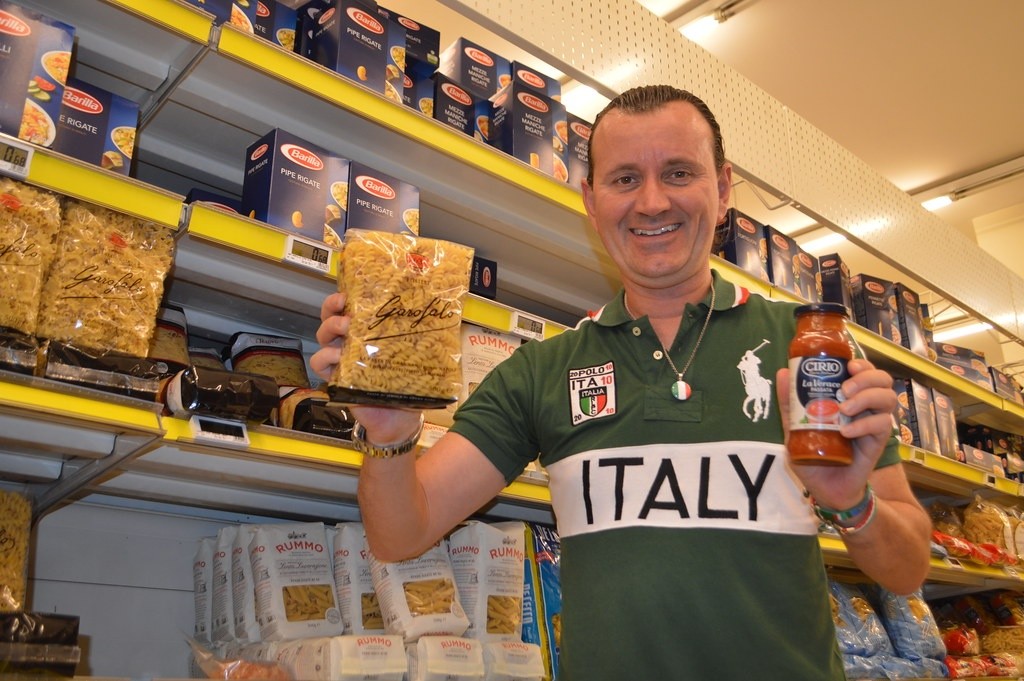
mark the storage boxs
[3,0,1024,491]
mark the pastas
[36,199,177,359]
[331,228,474,402]
[0,488,33,610]
[0,176,62,335]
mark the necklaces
[620,276,721,400]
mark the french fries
[931,492,1024,681]
[283,578,521,635]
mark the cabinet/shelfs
[1,0,1024,680]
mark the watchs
[348,417,427,459]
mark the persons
[310,85,933,680]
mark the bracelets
[799,490,876,537]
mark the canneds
[786,302,857,467]
